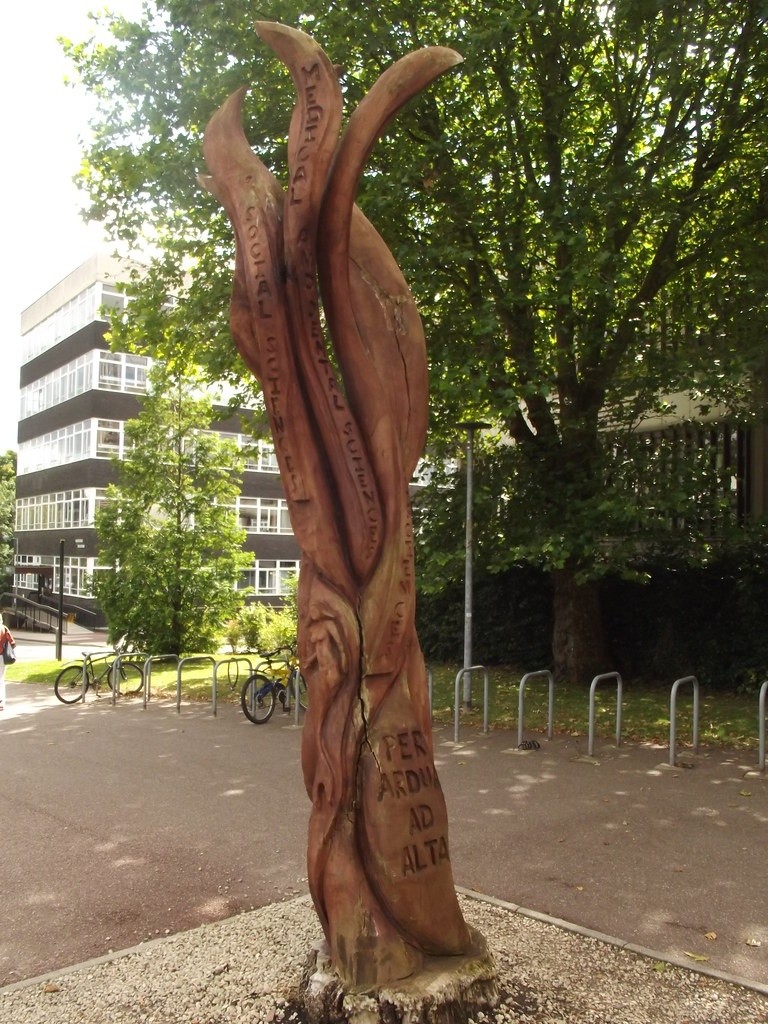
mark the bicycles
[54,651,144,705]
[241,643,307,724]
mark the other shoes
[0,706,4,711]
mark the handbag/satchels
[3,626,17,665]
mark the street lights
[455,422,494,711]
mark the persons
[38,573,45,604]
[0,614,16,711]
